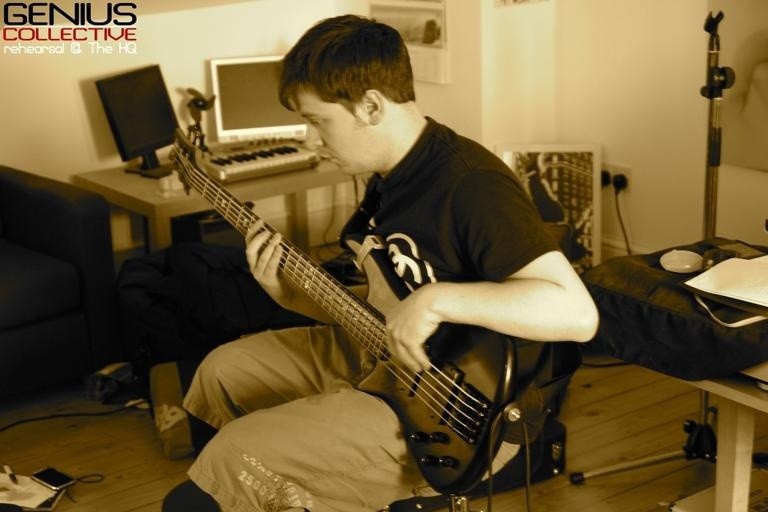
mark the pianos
[204,141,317,183]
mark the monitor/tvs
[94,63,179,177]
[209,55,308,143]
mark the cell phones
[31,467,77,490]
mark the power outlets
[610,165,633,195]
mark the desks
[647,364,768,511]
[71,137,404,264]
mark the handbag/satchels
[579,237,768,382]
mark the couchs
[0,148,128,404]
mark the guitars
[168,127,517,495]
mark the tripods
[570,7,768,483]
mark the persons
[162,16,600,512]
